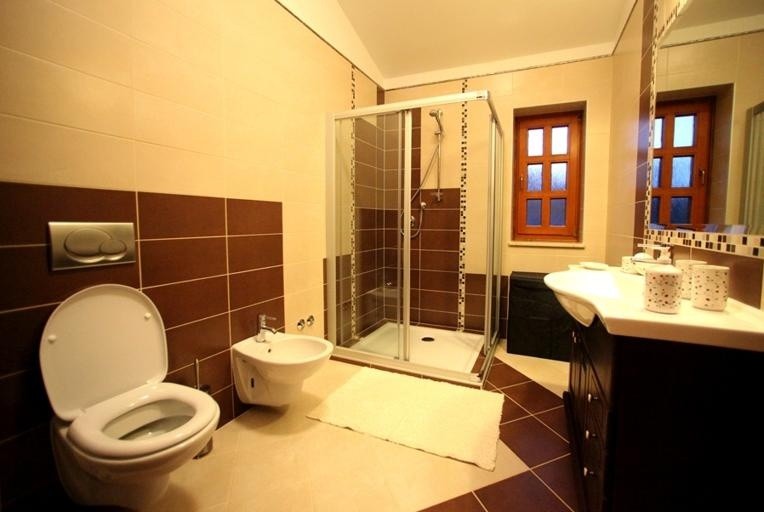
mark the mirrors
[648,0,763,236]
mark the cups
[686,264,731,312]
[642,266,685,315]
[674,258,707,300]
[621,256,637,273]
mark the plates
[580,261,608,270]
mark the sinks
[543,268,643,326]
[230,330,332,409]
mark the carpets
[304,366,504,472]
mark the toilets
[39,283,221,512]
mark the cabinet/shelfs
[562,314,617,511]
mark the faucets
[256,314,277,341]
[630,242,672,268]
